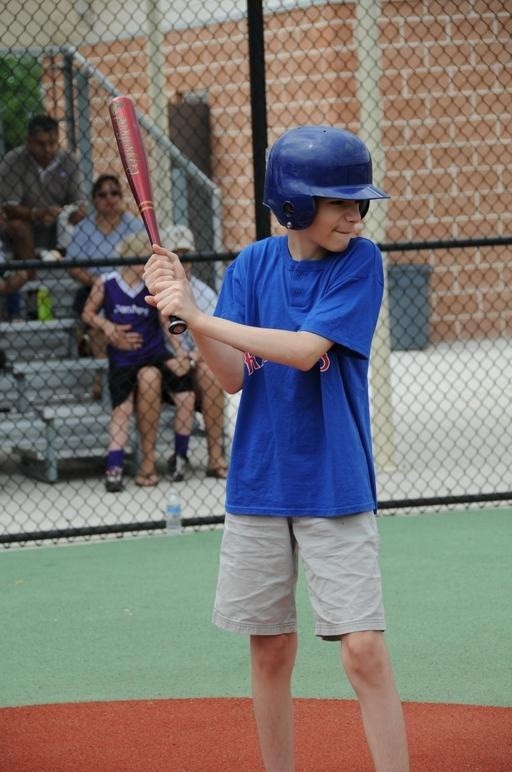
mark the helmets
[260,121,393,234]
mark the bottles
[26,283,54,321]
[165,488,182,536]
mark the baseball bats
[108,96,187,336]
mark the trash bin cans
[387,263,434,351]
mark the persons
[142,124,410,772]
[82,231,196,492]
[1,115,89,281]
[62,172,147,357]
[134,224,229,486]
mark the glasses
[96,189,121,199]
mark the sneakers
[205,458,230,479]
[168,451,190,484]
[105,462,124,491]
[134,466,159,488]
